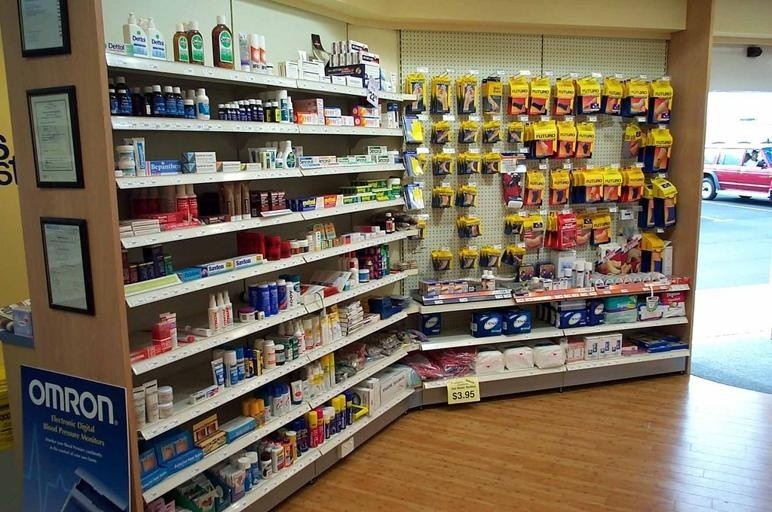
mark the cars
[702,144,772,203]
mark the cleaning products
[186,19,204,65]
[125,12,146,57]
[145,13,166,59]
[213,14,232,68]
[175,21,190,63]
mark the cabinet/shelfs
[410,283,690,411]
[0,0,418,512]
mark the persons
[743,149,763,167]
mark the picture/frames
[17,1,70,58]
[27,85,85,189]
[39,216,96,316]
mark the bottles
[107,11,396,495]
